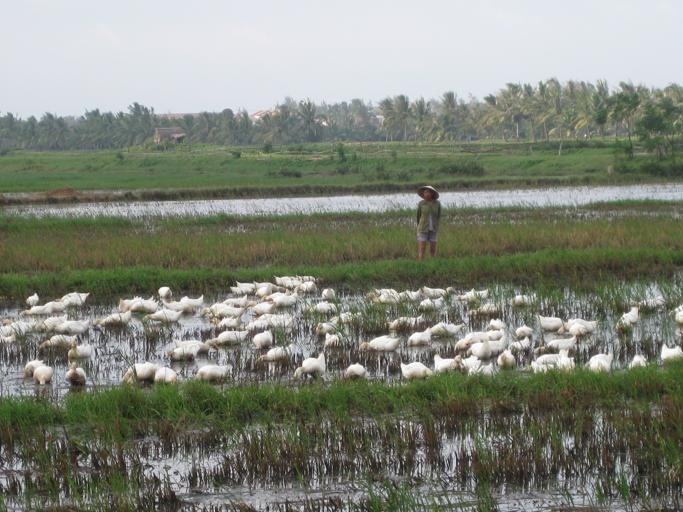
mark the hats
[417,184,440,201]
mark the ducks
[0,272,683,387]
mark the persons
[415,184,441,260]
